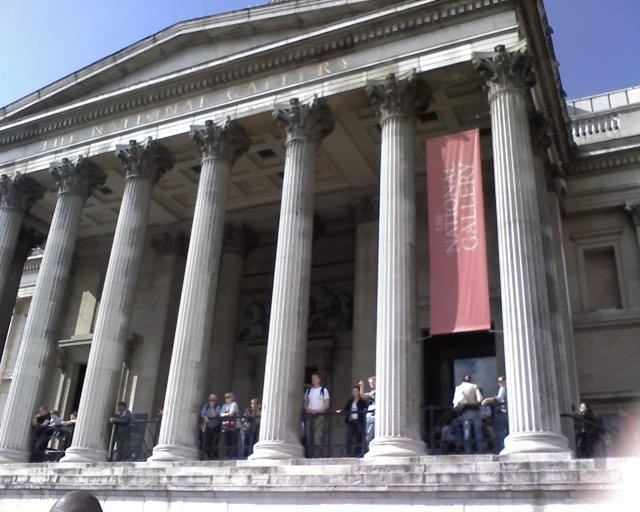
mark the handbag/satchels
[221,410,236,428]
